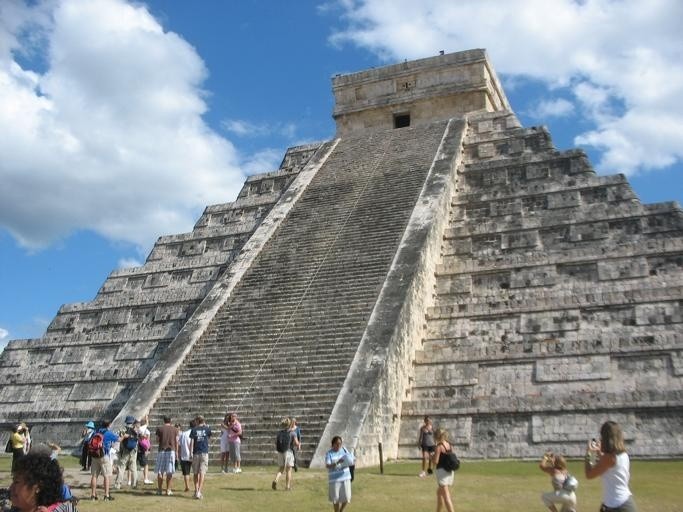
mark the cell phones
[592,438,596,448]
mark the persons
[583,420,638,512]
[539,449,578,512]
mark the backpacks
[122,426,137,451]
[85,429,108,458]
[276,430,293,453]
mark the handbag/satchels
[138,435,151,452]
[563,475,579,492]
[5,439,13,453]
[349,465,355,482]
[439,442,460,471]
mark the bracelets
[584,454,591,461]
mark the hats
[85,421,95,429]
[125,415,136,424]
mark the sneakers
[81,468,90,472]
[294,463,298,473]
[272,479,278,491]
[90,495,98,502]
[285,487,294,492]
[418,471,433,479]
[114,478,204,500]
[220,467,242,474]
[103,495,115,502]
